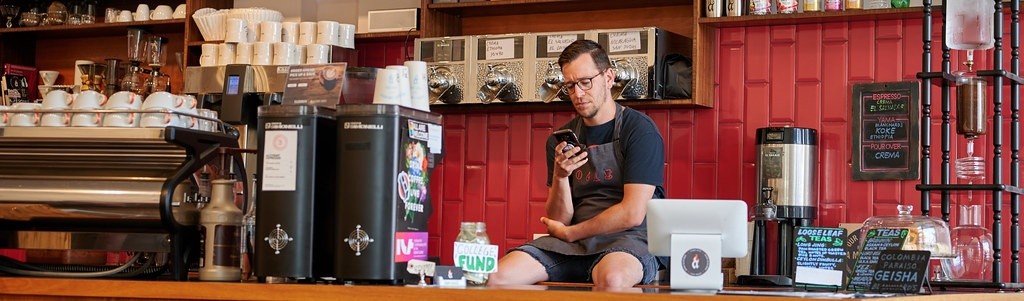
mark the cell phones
[552,129,586,162]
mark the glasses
[560,69,607,96]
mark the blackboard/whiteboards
[792,226,934,293]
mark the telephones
[852,81,920,181]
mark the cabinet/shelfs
[0,0,234,95]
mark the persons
[486,39,668,287]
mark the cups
[371,60,431,113]
[0,0,355,132]
